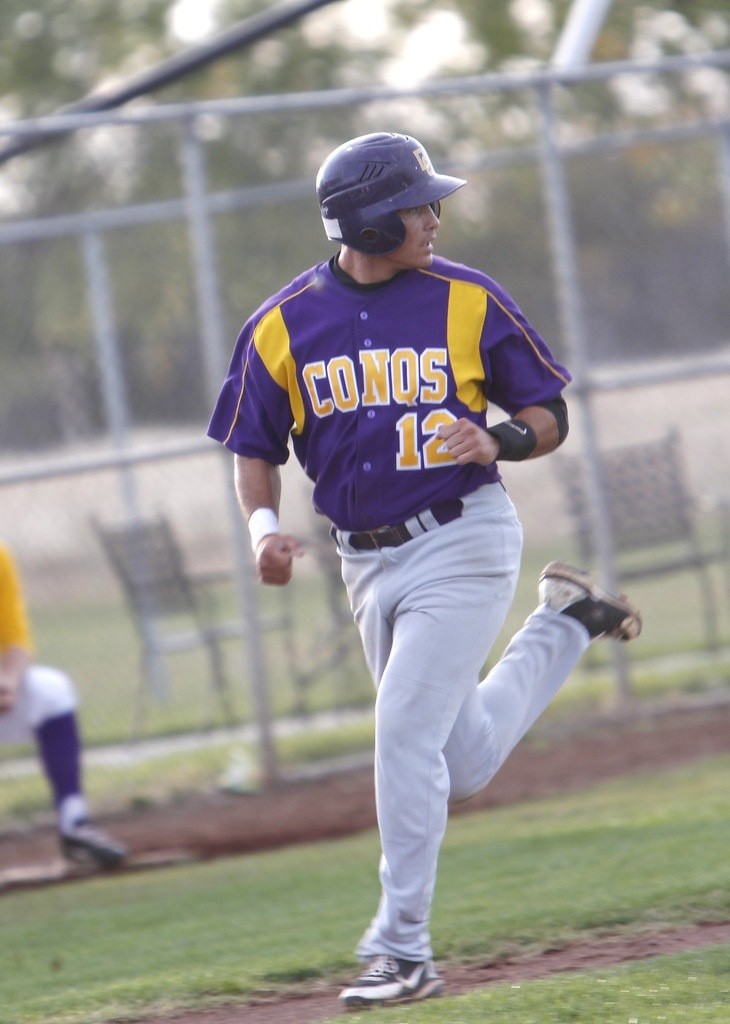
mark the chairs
[294,488,347,705]
[551,429,730,667]
[85,506,281,742]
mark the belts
[330,499,464,554]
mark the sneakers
[339,960,445,1011]
[538,563,641,645]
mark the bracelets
[484,418,538,466]
[247,507,286,554]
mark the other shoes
[63,822,125,866]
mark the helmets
[315,133,467,255]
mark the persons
[0,542,130,871]
[206,130,643,1014]
